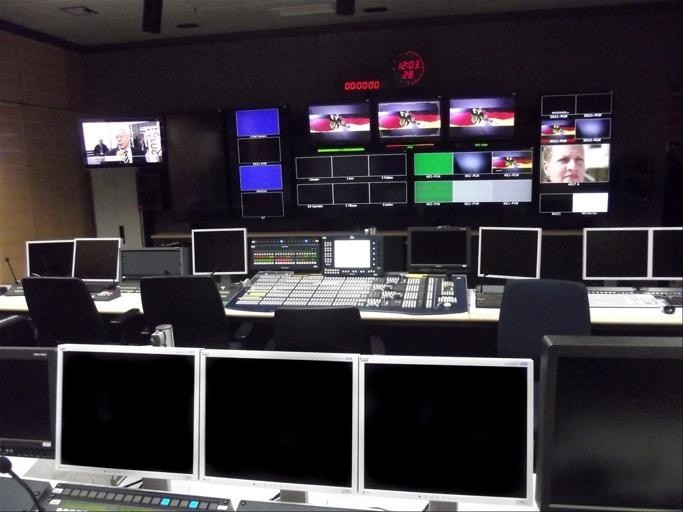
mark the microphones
[1,457,40,507]
[5,256,19,283]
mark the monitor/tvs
[575,118,611,143]
[191,227,248,302]
[581,227,651,283]
[541,119,575,145]
[539,94,576,116]
[0,346,54,460]
[448,95,519,148]
[413,150,533,178]
[200,347,357,495]
[576,93,612,115]
[377,94,443,147]
[536,333,683,507]
[54,342,200,480]
[321,233,385,277]
[538,191,609,213]
[294,153,408,182]
[414,180,533,207]
[78,118,166,170]
[307,97,374,152]
[476,227,543,280]
[357,354,533,507]
[406,227,473,277]
[119,246,190,293]
[237,108,285,216]
[25,240,75,278]
[540,144,611,185]
[296,182,408,209]
[650,227,683,282]
[73,237,122,301]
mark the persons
[92,129,147,164]
[540,144,596,183]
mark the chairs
[139,279,230,349]
[496,279,591,368]
[21,276,101,342]
[272,305,364,356]
